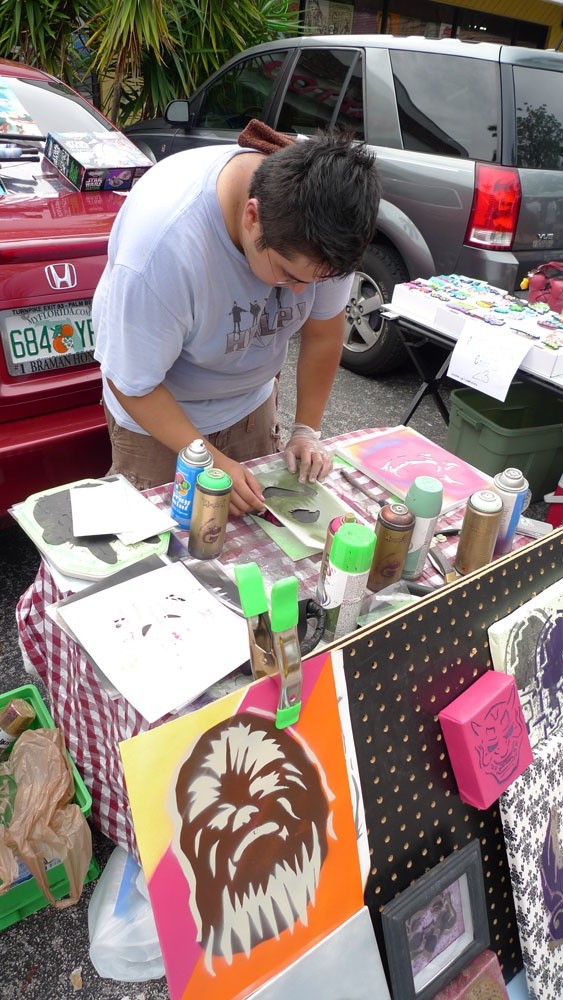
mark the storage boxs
[44,129,153,194]
[432,948,511,1000]
[440,669,536,810]
[445,381,563,503]
[51,190,129,219]
[392,274,562,380]
[0,685,102,934]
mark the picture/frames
[381,837,491,999]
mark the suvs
[118,32,561,372]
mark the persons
[88,134,385,514]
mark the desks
[379,302,563,428]
[15,424,540,865]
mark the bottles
[171,440,213,532]
[316,512,367,604]
[1,698,36,761]
[397,475,444,581]
[455,491,505,575]
[188,467,231,560]
[493,469,529,559]
[364,503,414,591]
[320,522,379,644]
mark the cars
[2,59,196,538]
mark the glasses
[259,219,334,288]
